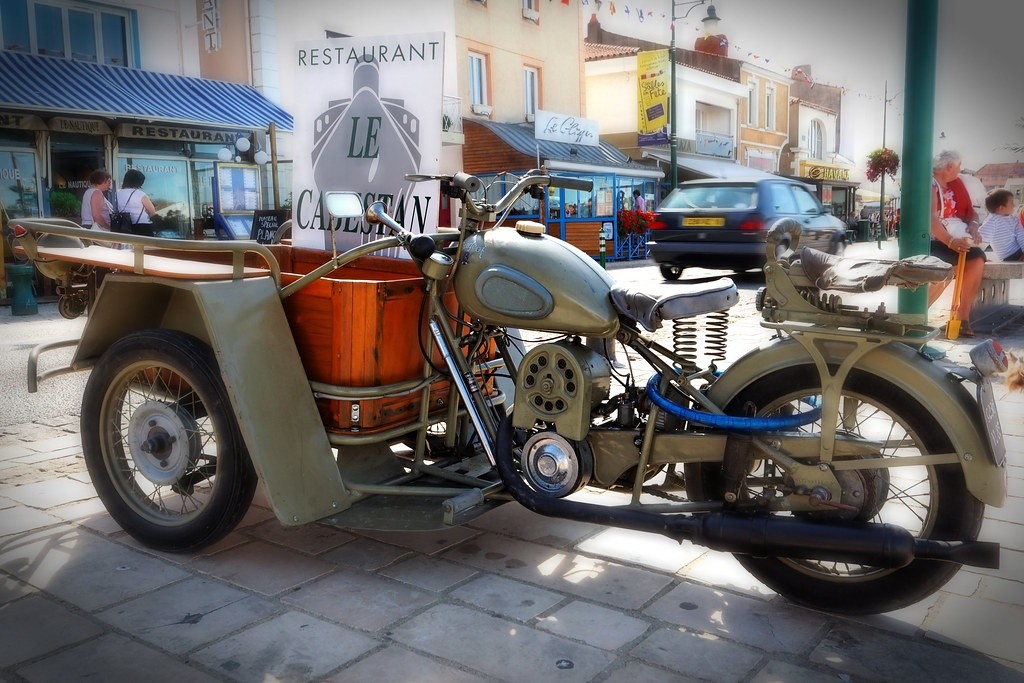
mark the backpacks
[109,189,144,234]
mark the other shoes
[959,320,975,338]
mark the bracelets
[970,220,980,227]
[948,236,956,248]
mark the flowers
[617,211,658,238]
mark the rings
[958,247,960,250]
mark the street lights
[668,1,723,191]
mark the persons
[565,189,644,218]
[930,151,986,336]
[81,171,113,231]
[849,204,893,242]
[978,189,1024,261]
[117,170,155,237]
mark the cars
[644,178,851,282]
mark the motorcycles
[4,167,1010,616]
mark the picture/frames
[250,209,289,242]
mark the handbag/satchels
[942,218,973,239]
[89,189,114,248]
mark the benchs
[939,260,1024,332]
[5,218,281,286]
